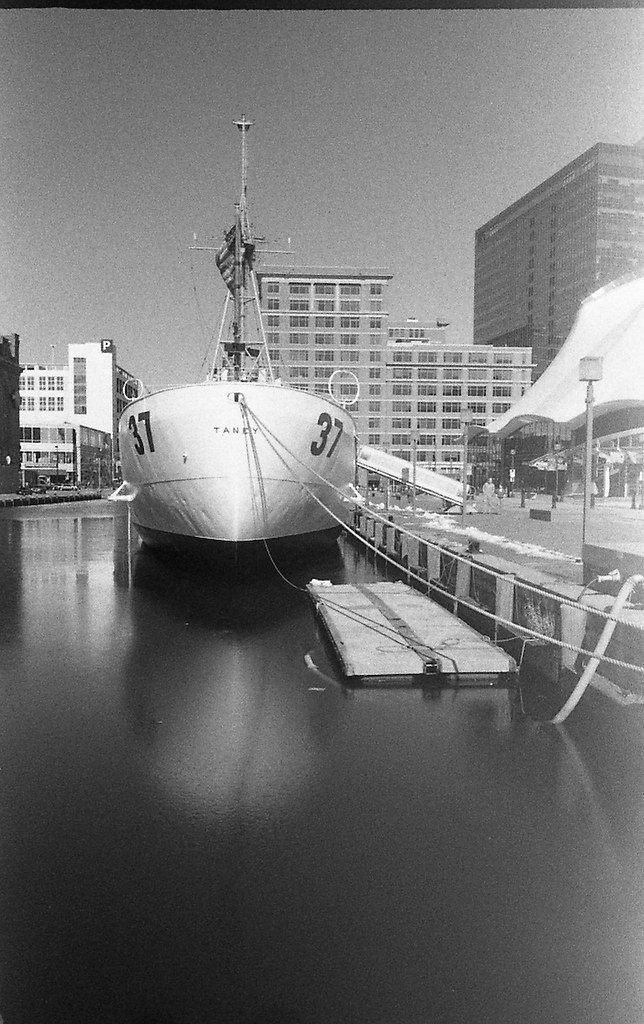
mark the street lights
[409,428,420,516]
[578,356,604,545]
[458,407,473,531]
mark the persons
[482,478,495,514]
[496,484,505,514]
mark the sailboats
[118,105,362,559]
